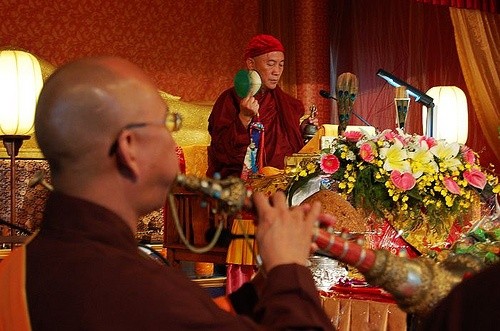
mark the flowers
[318,131,500,211]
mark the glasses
[107,111,182,156]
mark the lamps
[420,86,468,144]
[0,50,44,250]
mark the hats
[244,35,283,61]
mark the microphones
[376,69,433,107]
[319,90,371,126]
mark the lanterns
[420,86,468,146]
[0,50,44,135]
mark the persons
[206,34,320,180]
[25,55,338,331]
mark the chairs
[158,143,231,270]
